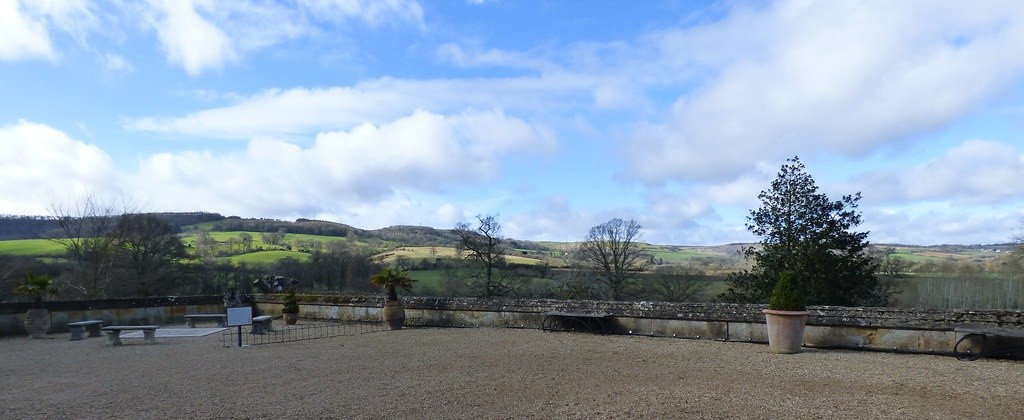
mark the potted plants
[369,267,420,330]
[282,289,299,325]
[763,269,811,356]
[15,271,60,339]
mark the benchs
[184,314,227,329]
[101,325,160,345]
[541,311,615,339]
[249,316,274,335]
[68,320,103,340]
[953,327,1024,362]
